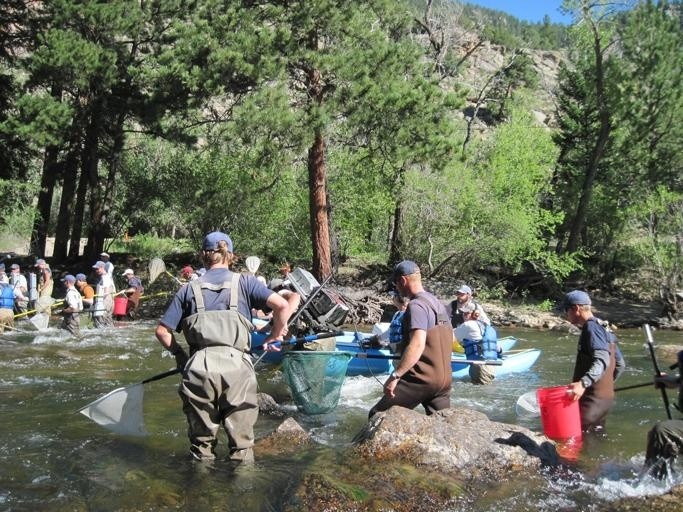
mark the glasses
[391,280,402,286]
[561,306,569,314]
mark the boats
[249,318,541,382]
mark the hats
[388,260,421,282]
[200,231,234,255]
[9,264,20,269]
[555,289,592,309]
[33,258,47,267]
[92,260,107,270]
[455,284,472,295]
[60,274,77,284]
[457,302,481,313]
[195,267,207,275]
[75,273,87,281]
[121,268,134,277]
[180,266,193,275]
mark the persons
[251,275,267,319]
[91,260,115,329]
[180,267,194,283]
[1,275,24,333]
[100,252,115,276]
[155,232,290,462]
[34,259,54,317]
[368,261,453,420]
[453,304,502,360]
[196,268,206,278]
[76,273,94,311]
[1,263,8,281]
[9,264,28,321]
[362,293,410,370]
[645,349,682,482]
[446,285,490,328]
[60,275,83,335]
[562,291,626,426]
[117,268,142,322]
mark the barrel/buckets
[535,385,582,439]
[113,292,128,316]
